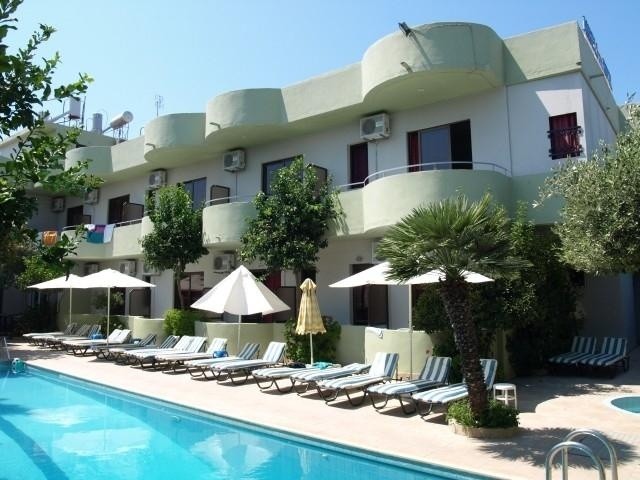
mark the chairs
[410,358,499,417]
[317,352,399,407]
[21,303,373,394]
[547,335,601,377]
[367,355,453,415]
[578,335,631,379]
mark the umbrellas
[294,277,329,367]
[69,267,157,349]
[25,273,83,329]
[189,264,293,357]
[327,253,497,383]
[27,384,107,429]
[298,446,320,479]
[50,393,150,459]
[188,427,285,480]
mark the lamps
[397,21,412,38]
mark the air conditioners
[81,187,98,207]
[358,113,393,142]
[119,260,136,277]
[144,169,168,190]
[221,148,246,173]
[49,197,65,213]
[141,261,162,277]
[79,263,100,275]
[370,240,388,265]
[210,253,235,274]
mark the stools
[492,382,518,410]
